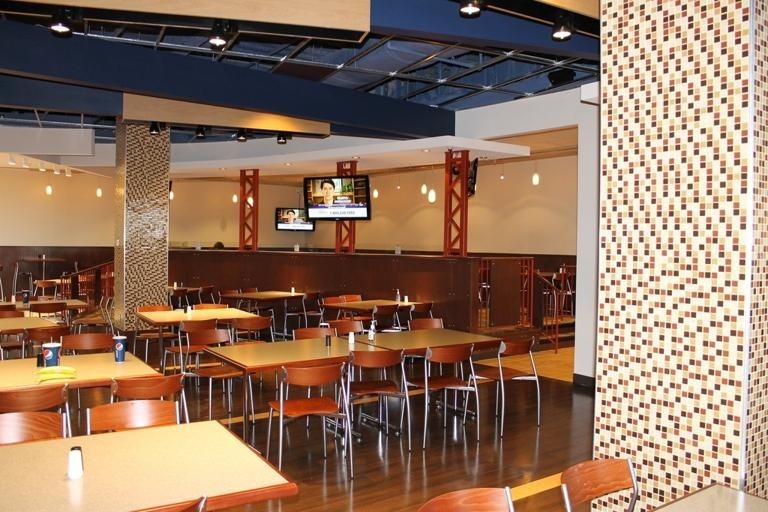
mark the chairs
[1,411,67,449]
[180,326,254,420]
[292,326,338,428]
[560,458,639,511]
[2,381,72,439]
[3,257,579,380]
[336,348,413,453]
[462,335,541,438]
[406,348,462,449]
[418,485,515,510]
[108,370,184,425]
[180,494,208,511]
[404,342,482,449]
[85,398,180,436]
[263,361,355,479]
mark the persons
[284,210,296,223]
[314,180,338,207]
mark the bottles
[396,288,408,303]
[326,335,331,346]
[348,332,354,343]
[37,353,43,367]
[395,244,401,254]
[68,446,84,481]
[183,304,191,314]
[368,321,376,340]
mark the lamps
[195,125,204,138]
[552,8,574,43]
[460,2,481,15]
[51,8,72,34]
[236,128,246,140]
[208,21,231,48]
[277,132,286,144]
[149,121,160,135]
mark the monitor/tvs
[303,174,372,221]
[274,207,315,231]
[468,157,478,196]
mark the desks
[648,483,768,512]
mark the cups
[42,343,62,367]
[113,335,127,364]
[294,244,299,251]
[22,289,30,304]
